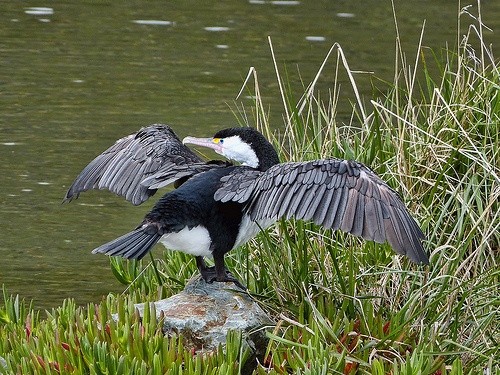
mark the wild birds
[59,123,429,291]
